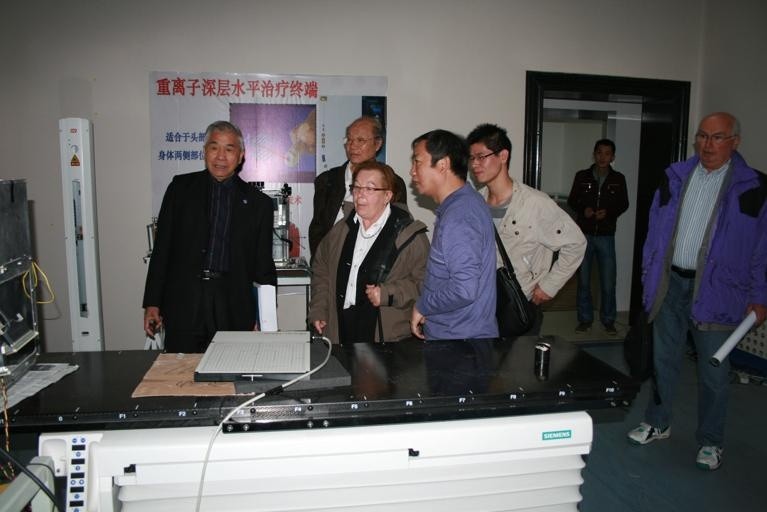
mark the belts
[670,265,696,279]
[198,270,223,281]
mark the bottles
[535,366,549,381]
[535,342,551,365]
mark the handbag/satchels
[496,266,535,337]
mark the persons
[326,344,407,401]
[567,138,629,336]
[309,160,431,344]
[309,115,407,258]
[409,129,500,342]
[465,122,588,335]
[141,120,277,354]
[626,112,766,472]
[423,341,511,396]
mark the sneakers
[626,422,672,446]
[695,445,727,471]
[604,323,618,335]
[576,322,592,333]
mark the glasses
[694,132,737,145]
[468,152,496,165]
[349,184,386,197]
[342,136,375,149]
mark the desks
[276,274,312,331]
[0,335,641,512]
